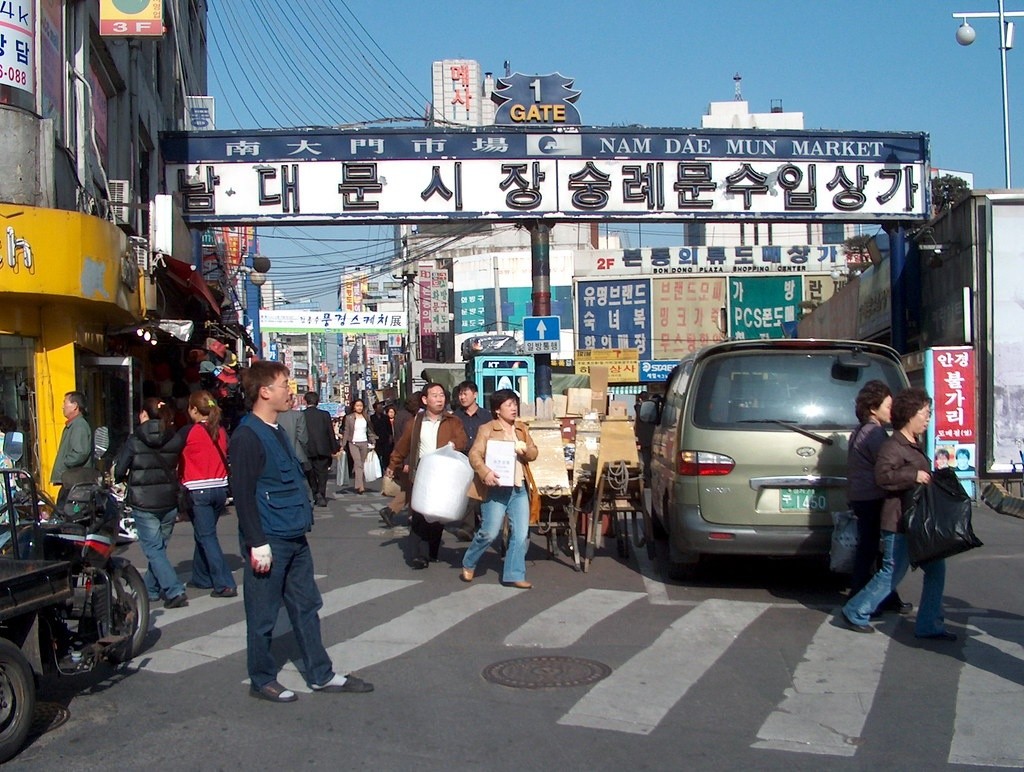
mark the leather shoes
[210,586,238,597]
[413,557,429,569]
[379,507,393,526]
[185,580,209,589]
[164,593,189,609]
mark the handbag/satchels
[175,485,192,521]
[905,468,984,567]
[363,450,382,482]
[831,509,859,573]
[336,451,349,486]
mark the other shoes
[918,632,958,641]
[457,526,474,542]
[839,609,874,633]
[881,590,913,614]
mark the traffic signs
[522,316,561,354]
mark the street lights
[953,0,1024,190]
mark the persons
[228,360,375,703]
[839,388,958,641]
[934,448,975,471]
[51,391,93,520]
[278,384,496,567]
[115,398,190,608]
[178,391,239,597]
[847,379,914,618]
[461,388,538,587]
[633,391,663,489]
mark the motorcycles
[0,426,150,763]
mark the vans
[639,338,923,584]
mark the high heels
[503,581,532,589]
[463,567,474,580]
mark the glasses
[267,383,290,389]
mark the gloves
[250,544,272,574]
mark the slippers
[324,674,374,693]
[251,680,298,702]
